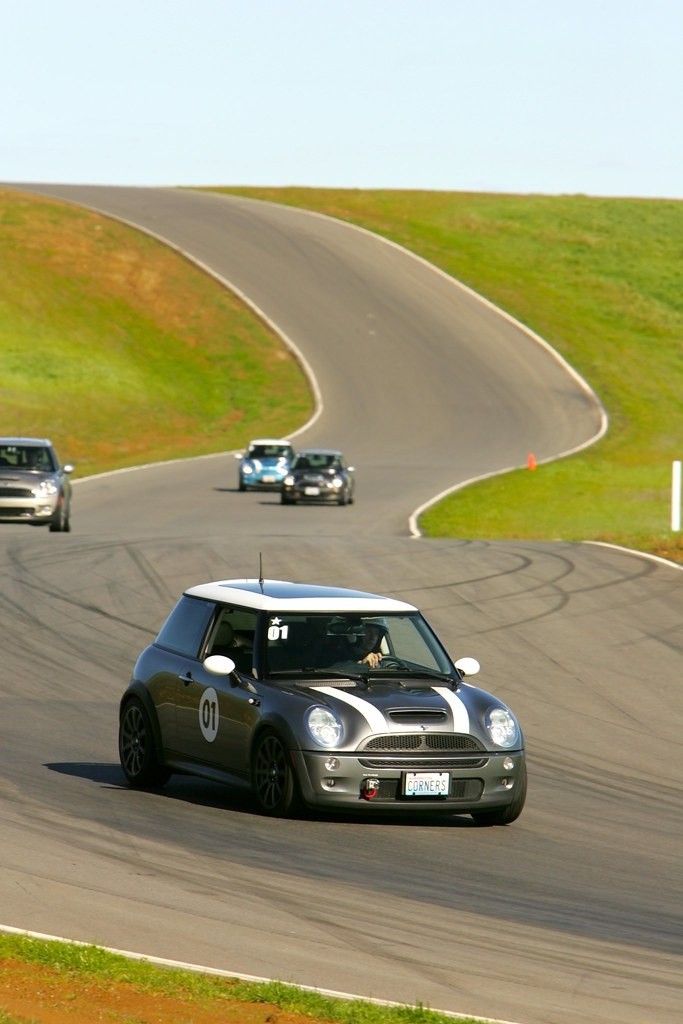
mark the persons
[29,450,42,465]
[327,456,338,467]
[343,618,389,669]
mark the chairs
[278,447,289,458]
[207,621,249,675]
[275,621,304,642]
[250,447,265,458]
[232,629,256,654]
[295,458,311,470]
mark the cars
[237,439,297,491]
[0,436,76,531]
[281,449,358,506]
[115,575,526,826]
